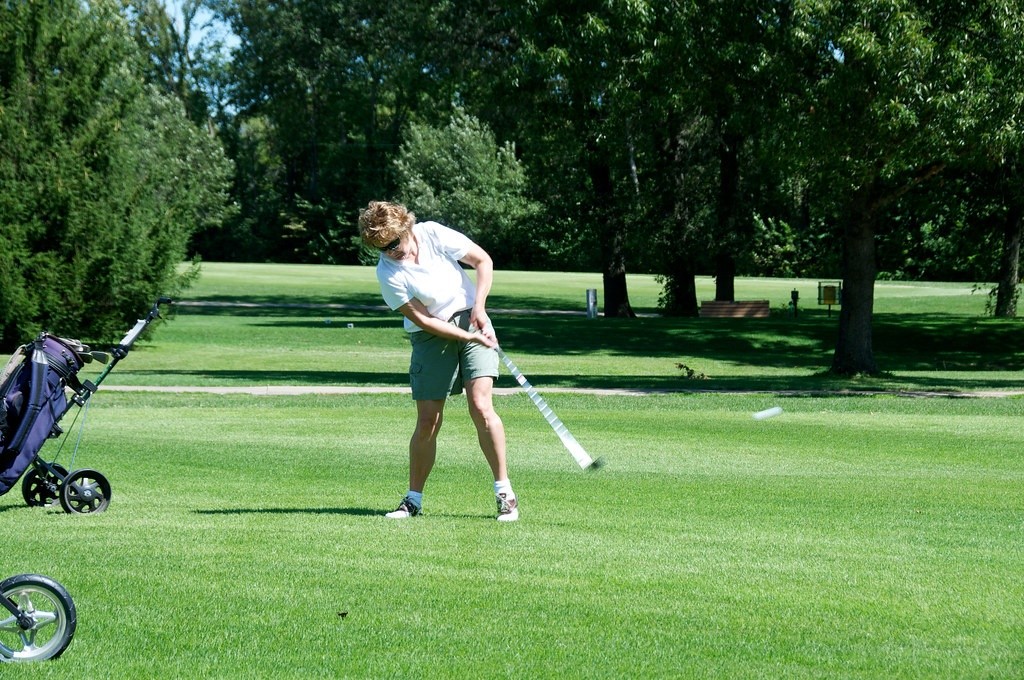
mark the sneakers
[385,496,423,519]
[495,492,519,522]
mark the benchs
[696,300,771,318]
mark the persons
[358,198,521,524]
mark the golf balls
[752,406,784,421]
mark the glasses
[372,235,401,252]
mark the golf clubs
[468,313,594,470]
[57,337,111,365]
